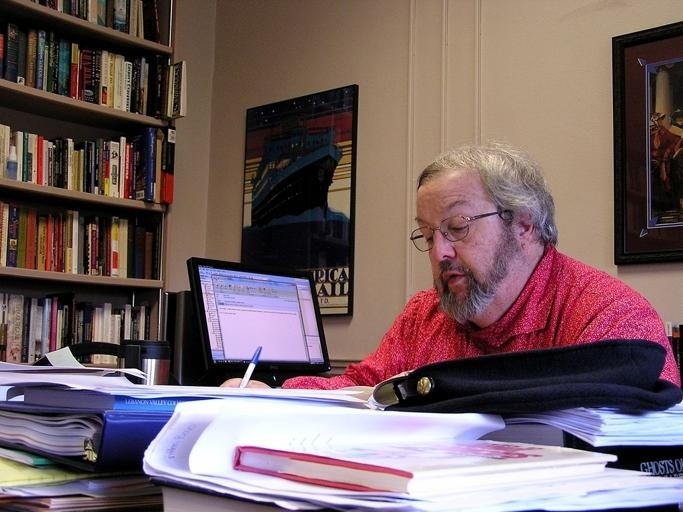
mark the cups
[121,340,171,385]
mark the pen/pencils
[239,346,261,389]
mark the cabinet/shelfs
[0,0,178,383]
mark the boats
[251,125,342,224]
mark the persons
[216,141,682,404]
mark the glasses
[410,209,513,253]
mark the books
[31,0,161,44]
[70,291,150,385]
[0,24,189,120]
[1,292,68,364]
[1,200,161,280]
[77,476,154,493]
[5,388,195,413]
[0,125,176,205]
[230,440,620,502]
[0,445,55,469]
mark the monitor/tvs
[188,257,331,390]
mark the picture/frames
[612,21,683,267]
